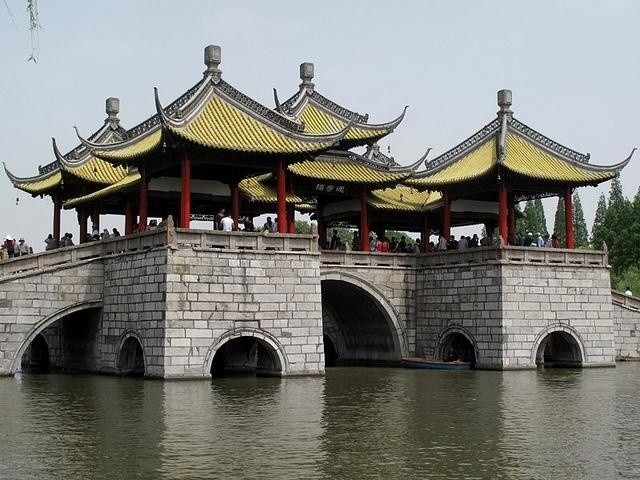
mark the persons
[625,287,632,296]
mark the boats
[398,356,473,370]
